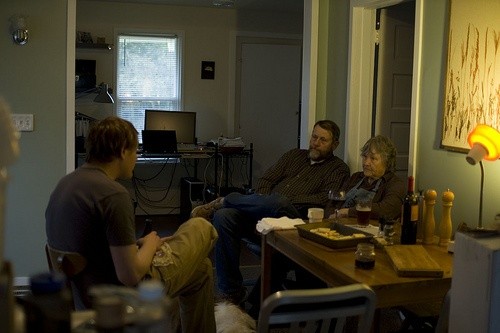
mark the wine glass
[328,190,346,223]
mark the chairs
[42,244,110,312]
[257,283,378,333]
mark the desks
[260,218,453,308]
[137,148,213,209]
[445,231,500,333]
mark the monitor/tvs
[145,109,196,145]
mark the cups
[356,200,372,227]
[308,208,324,224]
[383,224,396,245]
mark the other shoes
[190,196,225,221]
[225,287,249,303]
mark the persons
[191,119,405,304]
[45,116,219,333]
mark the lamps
[466,125,500,238]
[94,83,114,104]
[10,15,29,46]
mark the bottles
[355,244,376,270]
[400,177,420,244]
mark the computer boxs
[181,176,203,223]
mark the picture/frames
[438,0,500,154]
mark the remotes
[356,241,376,267]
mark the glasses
[310,135,333,144]
[360,153,390,162]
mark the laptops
[142,130,182,158]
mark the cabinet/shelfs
[192,137,254,198]
